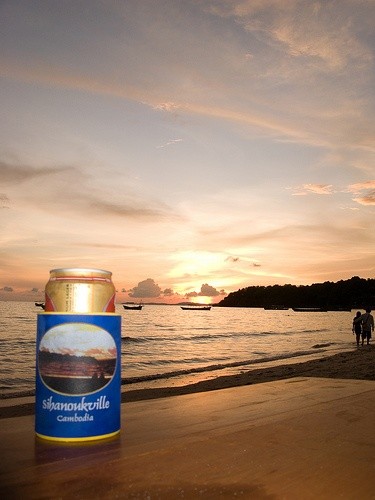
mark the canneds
[44,267,115,313]
[34,312,121,443]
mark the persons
[351,310,363,350]
[91,370,105,381]
[353,307,374,346]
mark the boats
[34,302,45,309]
[291,307,328,312]
[122,304,142,310]
[264,305,288,310]
[181,306,211,310]
